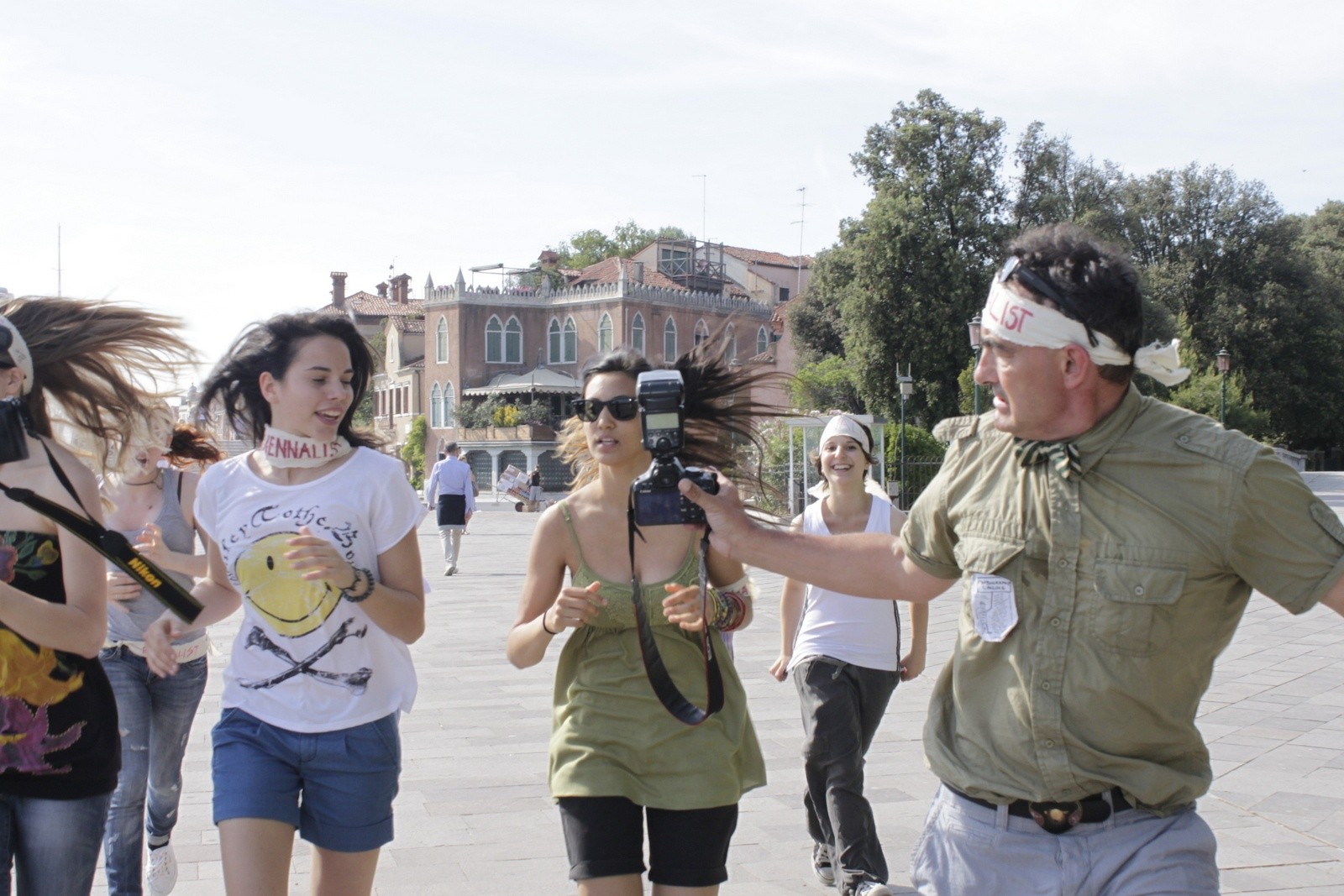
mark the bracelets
[338,565,362,593]
[542,607,558,636]
[705,586,746,632]
[342,567,375,602]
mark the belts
[940,779,1132,835]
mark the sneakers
[146,846,179,895]
[443,564,459,576]
[810,840,837,886]
[849,881,891,896]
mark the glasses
[571,395,639,422]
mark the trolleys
[485,482,544,513]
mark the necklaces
[119,467,162,491]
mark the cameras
[632,368,716,526]
[0,398,31,466]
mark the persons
[679,225,1344,896]
[768,415,930,896]
[0,292,205,896]
[503,318,807,896]
[427,441,478,576]
[528,464,542,513]
[83,393,204,896]
[141,313,429,896]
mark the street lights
[1217,346,1231,427]
[966,312,983,414]
